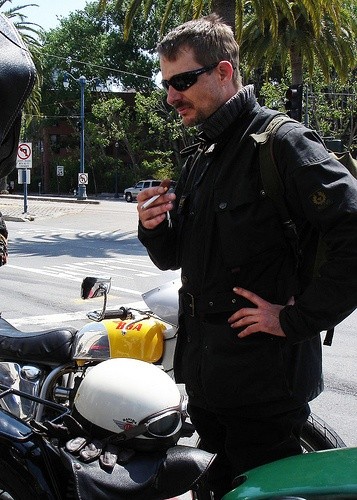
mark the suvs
[123,178,162,203]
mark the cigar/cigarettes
[141,187,169,209]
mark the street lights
[76,74,88,201]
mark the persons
[136,12,357,492]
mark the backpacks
[249,110,357,346]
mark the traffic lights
[284,88,300,120]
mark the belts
[178,288,257,317]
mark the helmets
[74,357,190,439]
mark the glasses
[103,383,188,442]
[162,59,237,92]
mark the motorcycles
[0,275,357,500]
